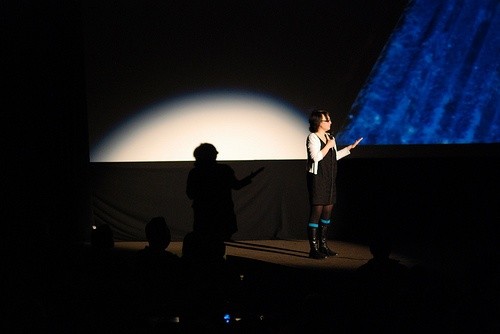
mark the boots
[306,222,328,260]
[317,219,338,256]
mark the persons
[1,215,500,334]
[306,108,363,260]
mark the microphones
[330,134,334,151]
